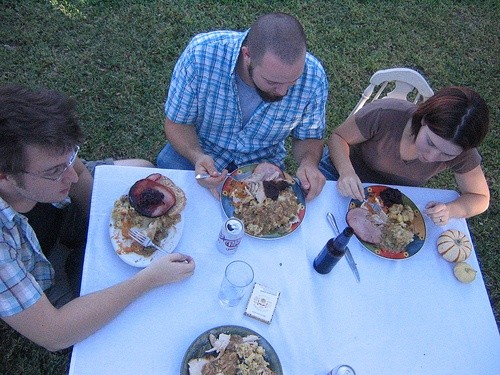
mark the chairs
[345,67,435,120]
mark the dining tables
[66,164,500,375]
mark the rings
[440,217,442,222]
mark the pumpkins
[454,262,477,283]
[437,229,472,263]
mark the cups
[217,260,255,307]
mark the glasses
[19,144,80,183]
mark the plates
[179,325,283,375]
[108,194,185,268]
[347,185,427,261]
[219,163,307,241]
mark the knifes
[327,212,360,282]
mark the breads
[145,173,185,217]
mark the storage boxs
[243,281,281,325]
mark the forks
[128,228,189,265]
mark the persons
[318,86,490,226]
[157,13,329,201]
[0,84,196,353]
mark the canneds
[218,217,243,256]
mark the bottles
[313,227,354,274]
